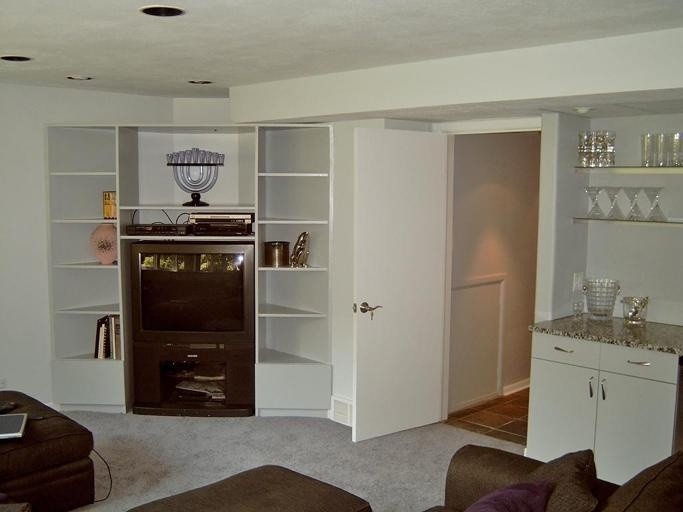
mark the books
[92,315,120,359]
[176,381,224,396]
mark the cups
[623,296,648,328]
[264,240,289,268]
[583,277,621,320]
[641,129,683,167]
[575,128,616,166]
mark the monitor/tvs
[132,242,254,343]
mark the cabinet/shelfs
[569,160,683,227]
[45,124,127,414]
[522,331,679,487]
[255,124,333,420]
[117,124,257,209]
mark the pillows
[464,448,600,512]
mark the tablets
[0,413,28,439]
